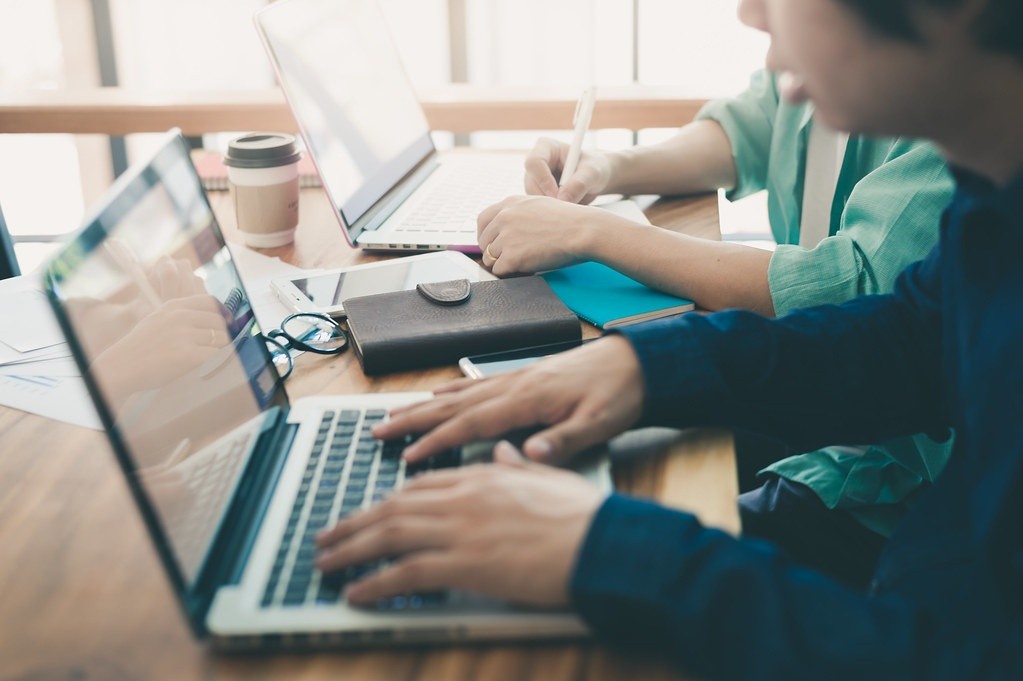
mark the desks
[0,148,743,681]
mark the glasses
[263,311,350,380]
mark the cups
[223,132,304,249]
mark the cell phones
[452,335,593,383]
[272,246,498,325]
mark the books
[536,262,696,330]
[342,276,582,375]
[189,146,323,191]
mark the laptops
[30,130,614,647]
[254,0,590,257]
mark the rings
[486,244,498,260]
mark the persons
[313,0,1022,681]
[476,68,957,591]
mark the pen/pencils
[560,90,602,189]
